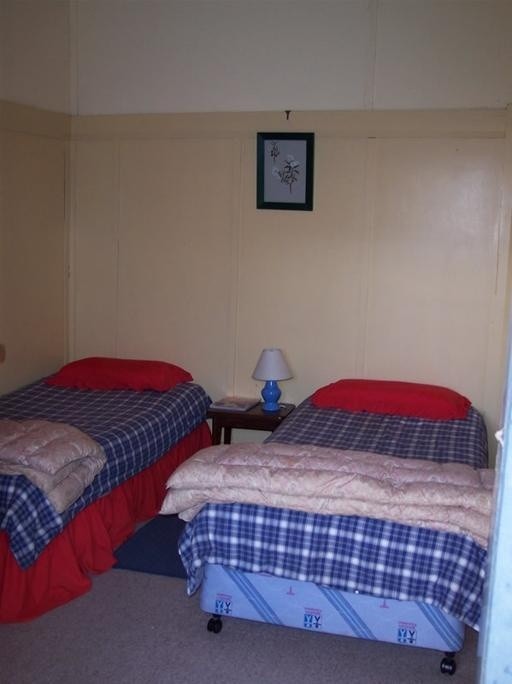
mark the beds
[159,400,495,676]
[0,377,211,629]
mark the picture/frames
[255,131,315,211]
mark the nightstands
[207,400,294,446]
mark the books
[210,396,260,412]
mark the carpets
[0,566,483,682]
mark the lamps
[250,346,293,412]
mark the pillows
[310,377,472,423]
[47,353,192,393]
